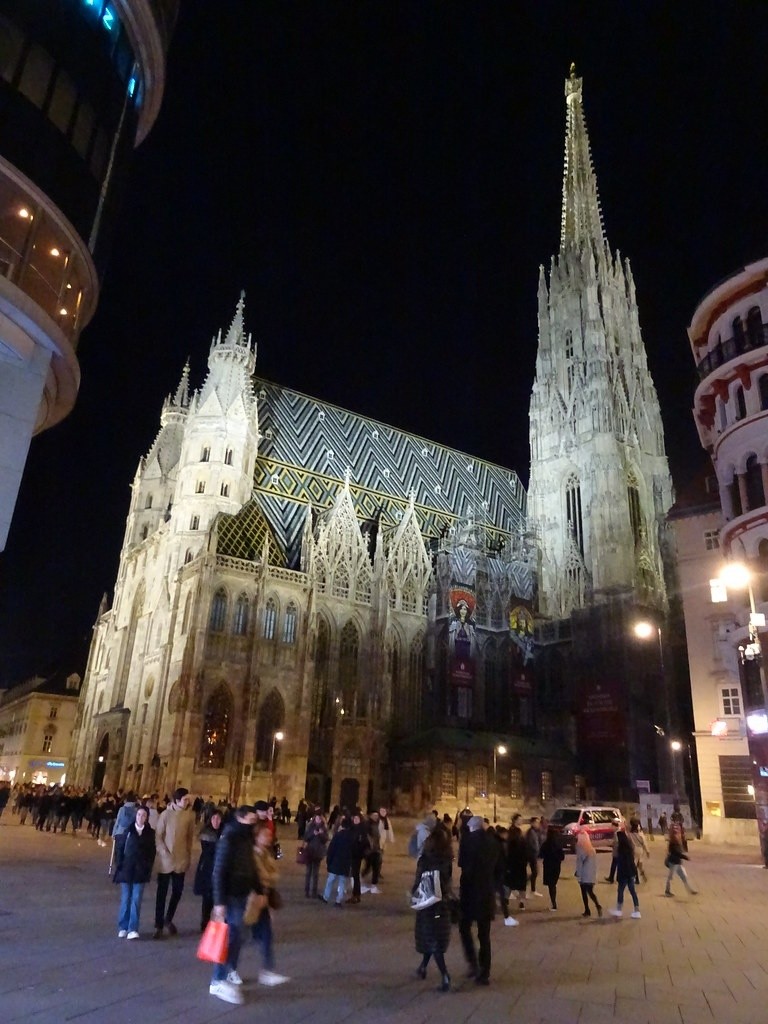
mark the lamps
[711,717,740,735]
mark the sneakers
[210,982,245,1005]
[258,970,288,987]
[118,930,128,937]
[127,932,139,940]
[227,971,243,985]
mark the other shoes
[442,975,450,990]
[153,928,160,939]
[474,978,488,985]
[464,965,478,977]
[496,873,698,919]
[164,923,177,934]
[504,916,520,926]
[307,883,383,907]
[416,967,426,979]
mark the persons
[254,800,273,832]
[524,816,543,897]
[295,800,394,907]
[217,799,232,815]
[193,809,225,933]
[648,808,700,896]
[0,781,116,838]
[109,788,135,835]
[112,793,138,872]
[629,817,650,885]
[192,794,204,824]
[115,805,156,938]
[201,796,213,823]
[604,818,642,919]
[270,797,276,808]
[209,804,285,997]
[576,829,602,917]
[141,794,160,829]
[280,795,292,824]
[254,827,282,918]
[411,809,528,991]
[155,788,197,939]
[267,803,273,815]
[539,828,565,911]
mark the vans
[547,807,627,854]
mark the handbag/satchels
[268,888,282,910]
[197,920,229,963]
[409,869,442,910]
[274,843,283,859]
[295,846,306,862]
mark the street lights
[494,746,505,823]
[268,732,283,804]
[727,533,768,713]
[638,624,688,851]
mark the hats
[467,816,482,829]
[253,801,269,811]
